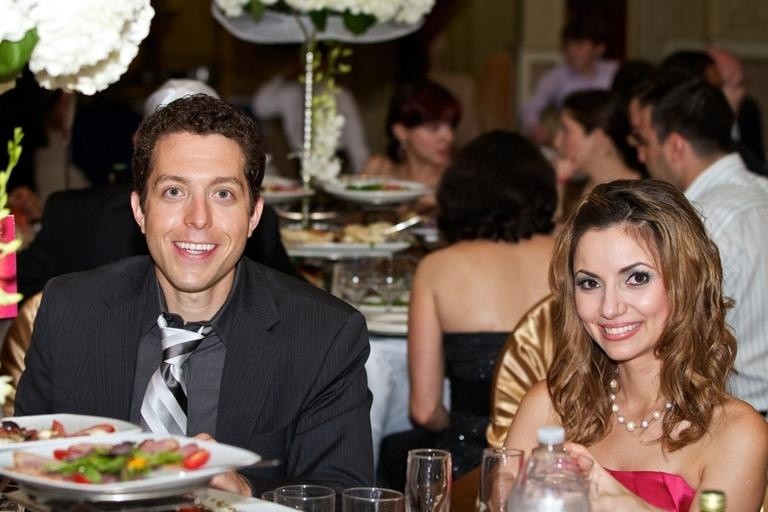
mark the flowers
[287,39,357,186]
[1,2,154,96]
[215,0,438,34]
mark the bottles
[698,490,726,512]
[530,427,566,457]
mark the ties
[135,313,216,439]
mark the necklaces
[604,367,678,432]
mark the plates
[1,412,142,450]
[256,173,438,261]
[1,433,263,501]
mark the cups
[506,448,593,512]
[262,444,526,512]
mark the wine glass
[341,264,408,319]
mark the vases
[292,11,317,233]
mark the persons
[13,89,379,511]
[475,176,768,512]
[617,67,768,430]
[1,14,765,314]
[378,125,575,489]
